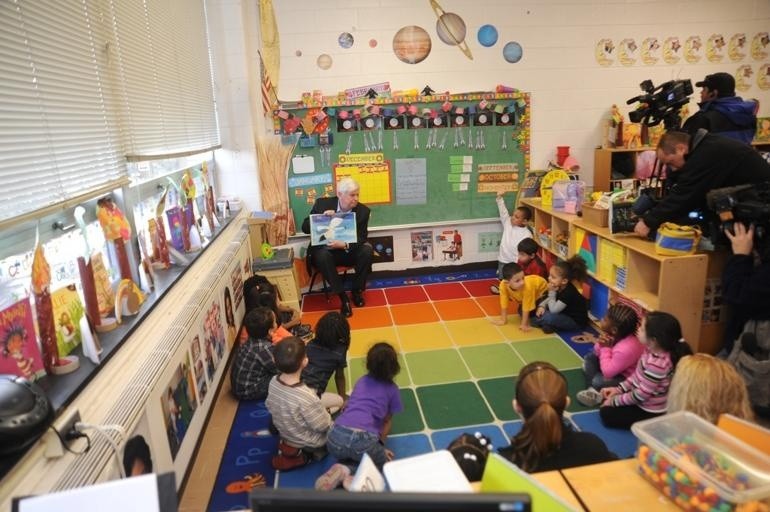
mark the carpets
[204,267,668,512]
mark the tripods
[648,116,683,200]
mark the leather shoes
[342,302,352,317]
[356,294,364,306]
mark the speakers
[0,373,57,454]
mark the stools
[308,252,365,304]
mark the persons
[411,229,465,265]
[223,284,237,347]
[303,180,374,317]
[266,338,340,472]
[635,131,769,240]
[492,186,589,334]
[686,72,758,142]
[579,304,753,434]
[314,343,400,492]
[721,222,770,359]
[230,274,314,401]
[498,361,619,469]
[300,311,353,416]
[446,432,494,479]
[120,337,215,477]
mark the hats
[695,72,735,92]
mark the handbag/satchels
[608,187,648,236]
[656,222,702,256]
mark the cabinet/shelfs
[520,197,709,355]
[594,139,770,197]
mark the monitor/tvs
[249,486,532,512]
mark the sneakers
[315,463,349,491]
[576,387,603,407]
[291,324,311,339]
[278,443,301,457]
[272,456,305,470]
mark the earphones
[710,92,716,98]
